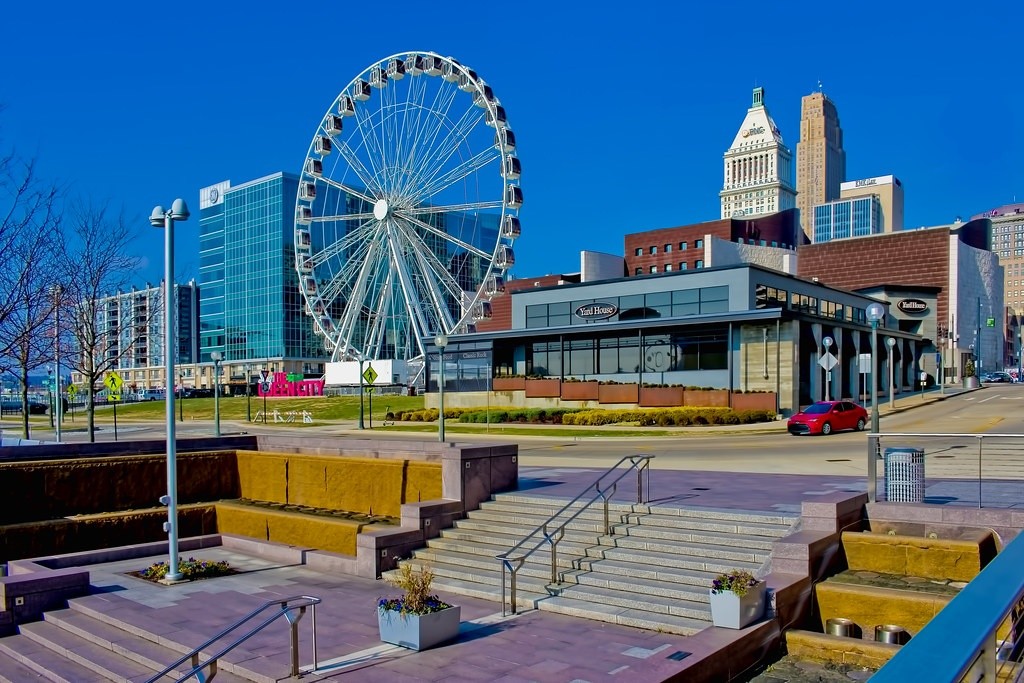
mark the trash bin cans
[883,447,926,502]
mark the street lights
[434,335,449,443]
[887,337,896,408]
[245,363,251,421]
[358,353,367,428]
[46,365,54,427]
[49,285,67,441]
[148,199,190,577]
[940,338,946,395]
[60,375,66,423]
[210,350,222,436]
[866,303,884,460]
[823,337,833,400]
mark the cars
[1,396,46,413]
[786,401,870,437]
[175,388,219,397]
[985,372,1013,382]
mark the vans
[138,389,167,401]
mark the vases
[710,578,766,629]
[378,600,461,651]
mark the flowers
[712,569,757,597]
[375,557,444,616]
